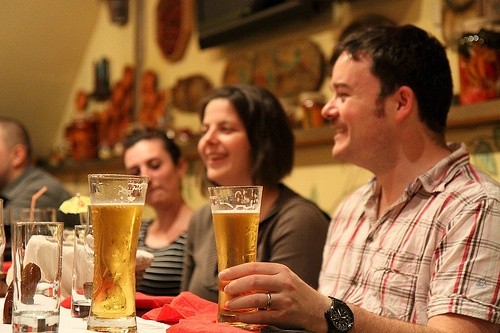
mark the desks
[0,295,179,333]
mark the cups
[0,198,64,333]
[87,173,149,333]
[207,185,263,329]
[72,225,94,318]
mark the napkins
[60,292,268,333]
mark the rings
[266,293,271,306]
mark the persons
[0,117,81,261]
[123,128,194,296]
[180,85,332,305]
[218,22,500,333]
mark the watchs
[324,296,354,333]
